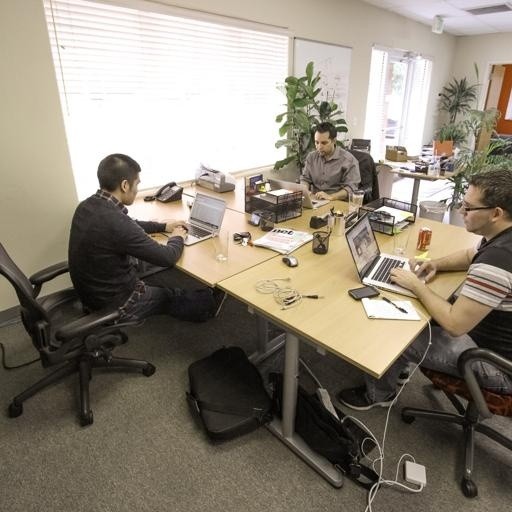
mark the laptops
[268,178,329,209]
[162,193,226,246]
[345,212,425,299]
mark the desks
[177,175,369,237]
[127,171,299,365]
[383,155,456,213]
[218,205,484,491]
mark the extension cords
[316,388,340,422]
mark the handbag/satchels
[184,346,276,446]
[274,371,383,493]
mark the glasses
[460,202,495,213]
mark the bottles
[335,210,345,237]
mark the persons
[300,123,361,199]
[68,153,230,325]
[336,170,512,413]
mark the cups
[349,193,361,214]
[353,189,365,208]
[394,223,411,253]
[212,229,229,261]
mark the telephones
[199,162,235,191]
[310,213,328,229]
[144,181,184,202]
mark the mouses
[283,256,298,267]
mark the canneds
[416,228,432,252]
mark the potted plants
[428,72,482,162]
[442,139,512,228]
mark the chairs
[0,242,157,428]
[400,346,512,498]
[348,150,379,205]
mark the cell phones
[349,286,380,300]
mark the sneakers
[214,288,226,317]
[338,388,398,411]
[397,367,410,387]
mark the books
[359,298,421,321]
[256,226,316,255]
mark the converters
[405,461,426,487]
